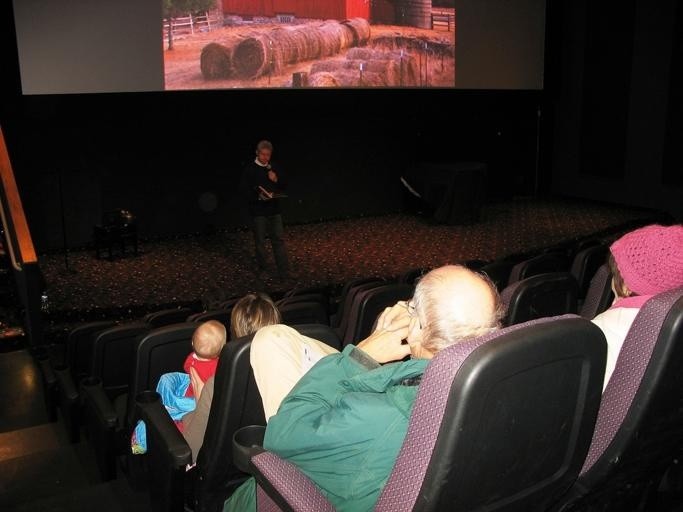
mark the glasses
[406,300,425,332]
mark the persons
[130,294,281,473]
[223,265,504,512]
[590,223,683,393]
[184,320,227,398]
[245,140,299,288]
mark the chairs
[67,209,683,512]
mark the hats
[609,225,683,296]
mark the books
[258,186,288,201]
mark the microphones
[266,164,278,183]
